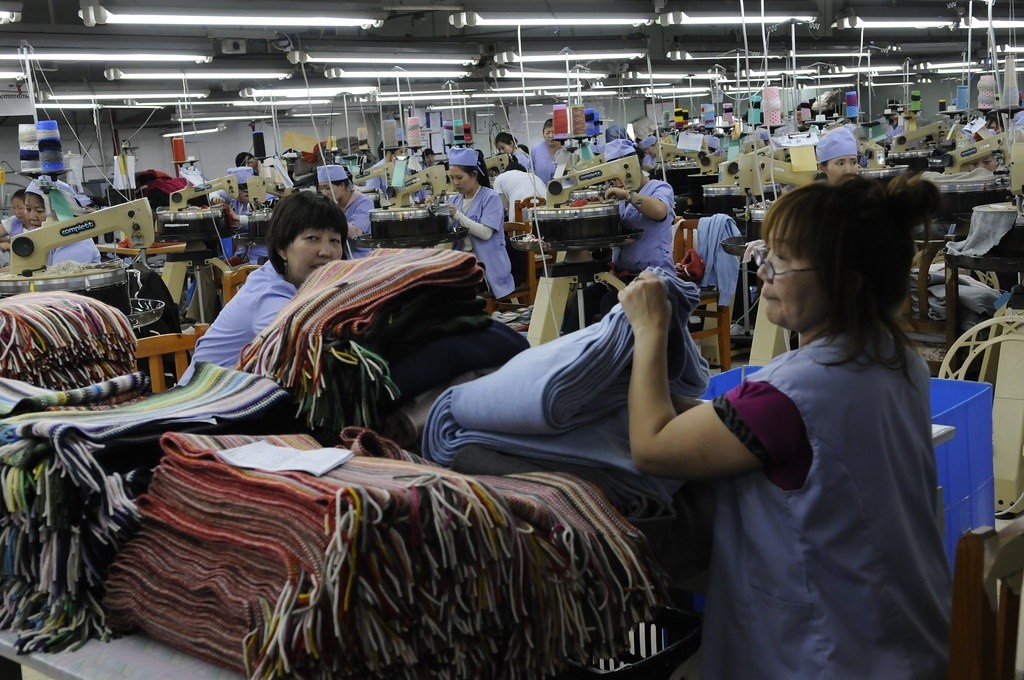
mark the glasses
[753,248,817,280]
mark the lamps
[0,0,1024,139]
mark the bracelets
[627,191,637,202]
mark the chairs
[671,215,742,373]
[482,220,536,316]
[898,234,959,380]
[514,196,558,270]
[131,323,210,391]
[224,264,262,306]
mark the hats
[448,147,479,166]
[227,167,255,184]
[816,127,858,162]
[25,174,73,199]
[604,139,636,161]
[640,136,657,148]
[317,165,348,182]
[708,137,720,149]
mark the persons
[619,175,952,680]
[366,130,408,192]
[605,126,658,174]
[420,148,436,169]
[970,109,1024,170]
[317,165,374,259]
[816,127,856,184]
[0,174,101,267]
[886,112,903,140]
[757,128,770,146]
[426,147,514,303]
[493,119,564,282]
[177,190,348,388]
[706,135,719,153]
[181,153,275,323]
[604,140,678,277]
